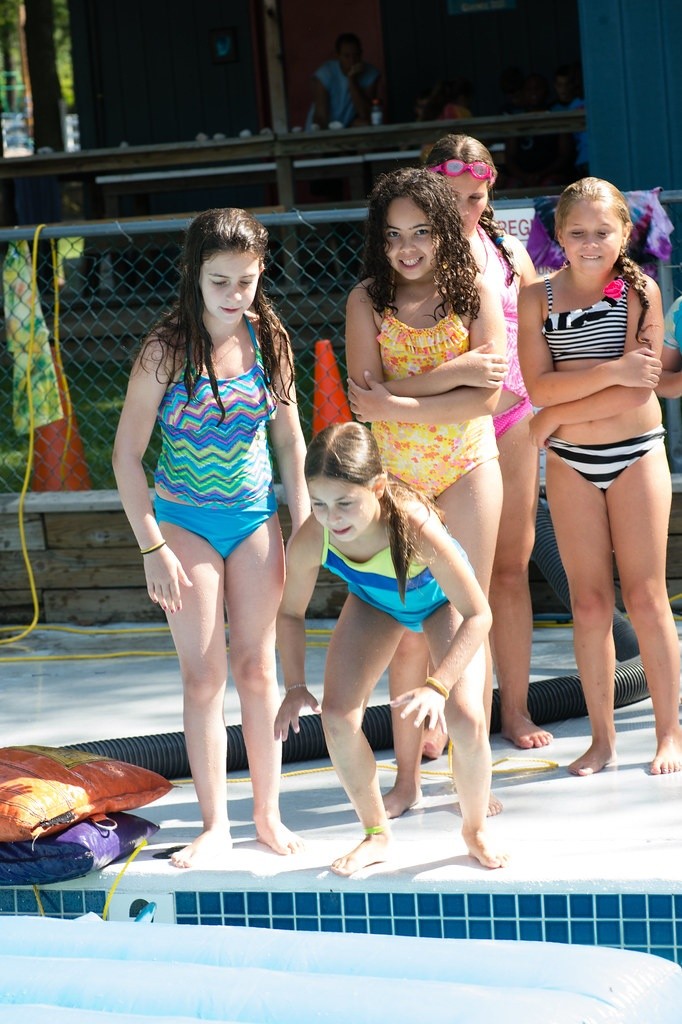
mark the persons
[517,177,682,775]
[305,33,589,202]
[273,421,512,877]
[344,165,509,819]
[420,134,554,759]
[112,208,312,868]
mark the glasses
[427,159,495,187]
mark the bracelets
[285,682,306,693]
[140,540,167,555]
[426,677,450,701]
